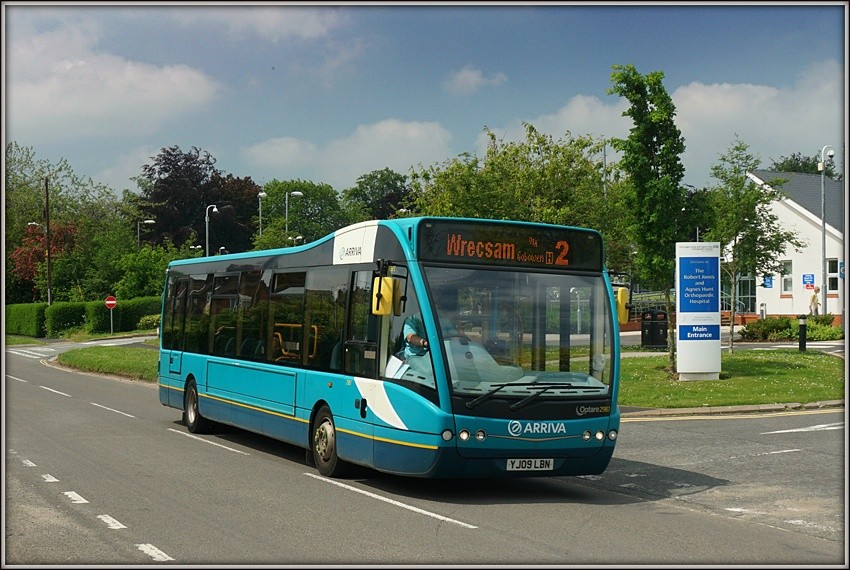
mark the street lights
[285,190,304,249]
[818,145,835,316]
[257,192,267,239]
[27,222,52,307]
[205,204,220,258]
[219,246,226,255]
[137,219,155,251]
[189,245,202,252]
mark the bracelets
[422,340,426,347]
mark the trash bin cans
[641,310,668,349]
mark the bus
[157,217,637,483]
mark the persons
[810,286,821,315]
[403,294,459,358]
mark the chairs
[241,338,259,356]
[224,337,236,353]
[330,341,364,370]
[214,335,234,354]
[254,341,282,356]
